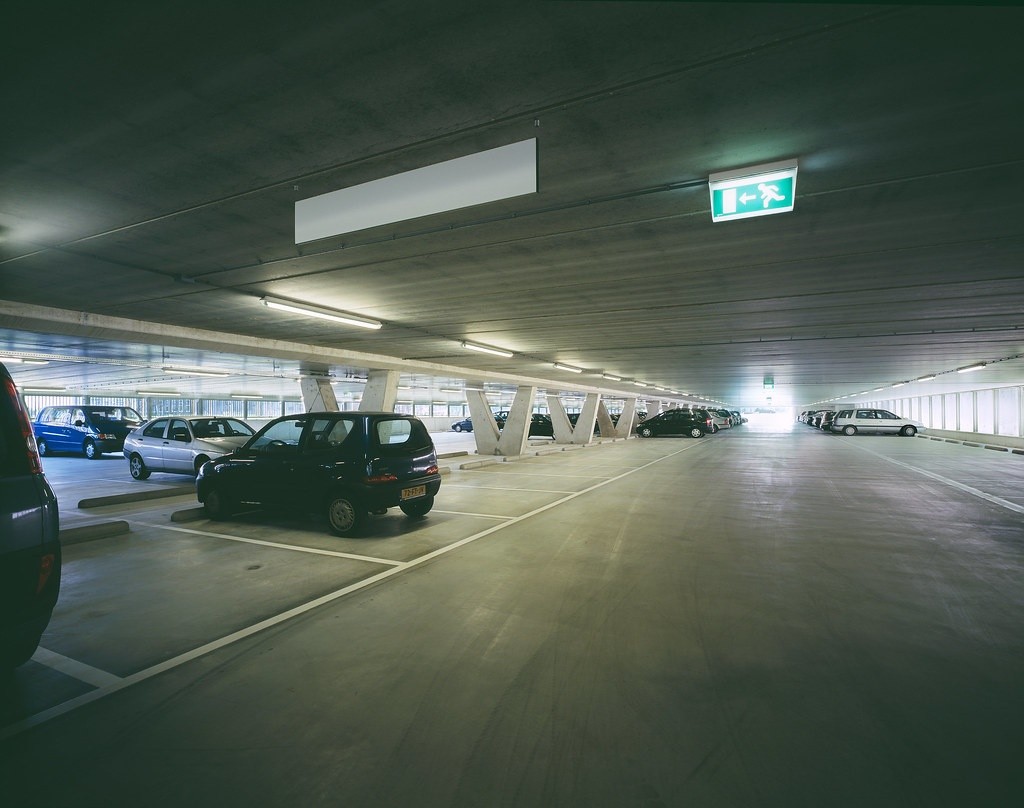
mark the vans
[32,404,149,460]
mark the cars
[451,417,473,433]
[123,415,281,481]
[1,362,62,671]
[493,411,647,441]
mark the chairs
[93,414,100,420]
[153,427,165,437]
[305,431,328,452]
[173,427,188,442]
[206,425,223,436]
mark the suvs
[799,409,880,431]
[829,408,926,436]
[635,408,749,439]
[195,411,441,538]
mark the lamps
[260,296,383,331]
[162,368,230,377]
[602,374,623,381]
[137,391,182,396]
[23,387,66,392]
[957,363,986,374]
[0,356,49,365]
[553,363,582,374]
[462,341,513,358]
[918,375,935,382]
